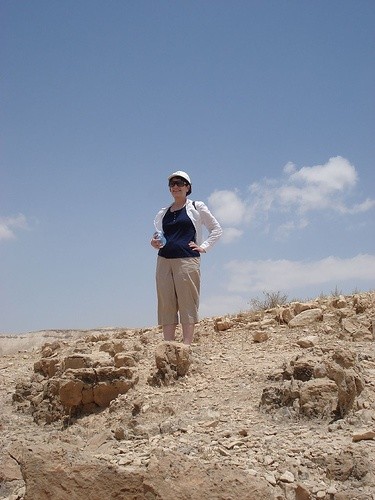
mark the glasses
[169,181,187,187]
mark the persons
[150,171,223,344]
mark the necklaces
[172,203,185,211]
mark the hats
[167,171,192,197]
